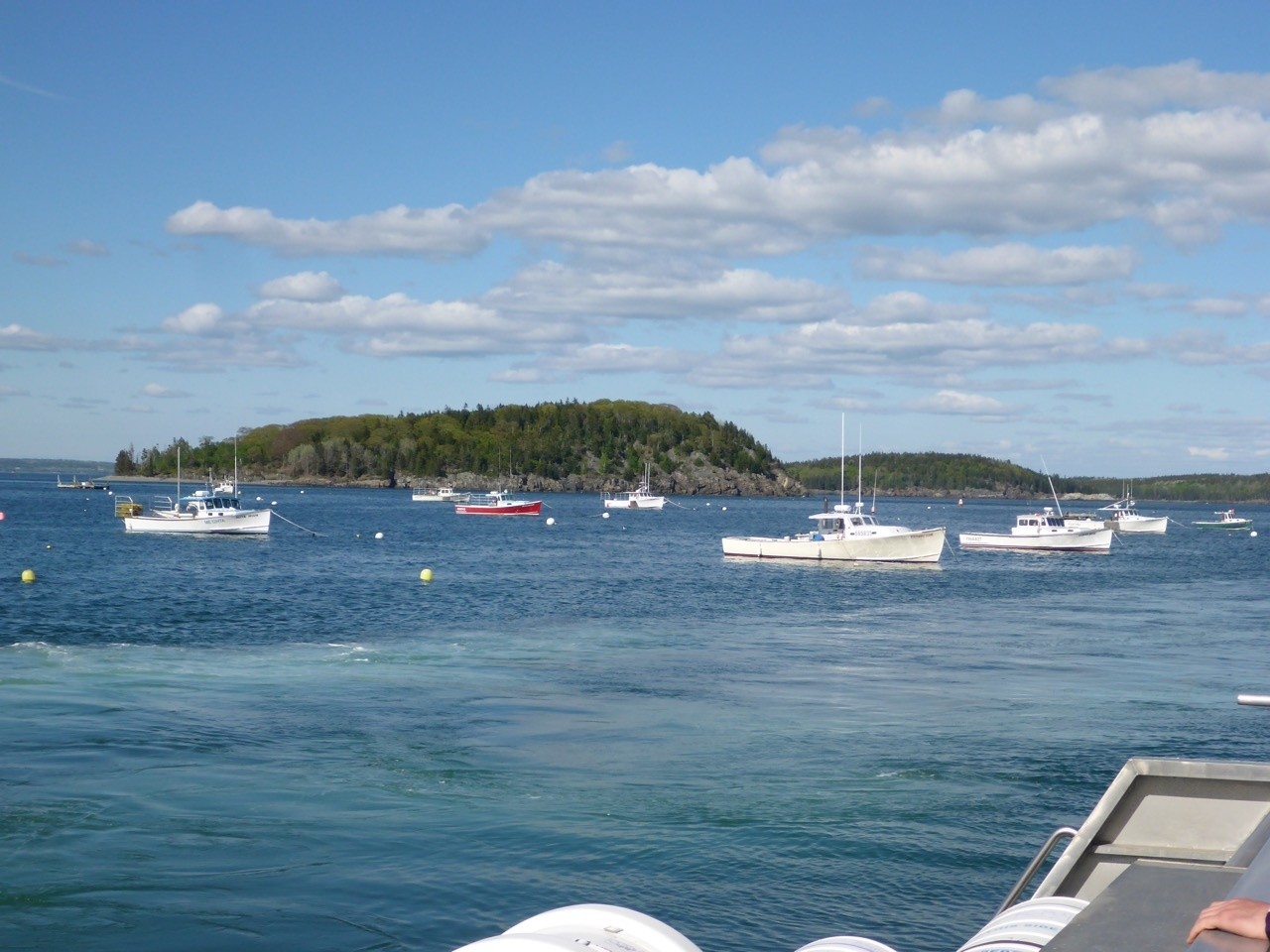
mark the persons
[1188,899,1270,943]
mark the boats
[1104,450,1168,534]
[412,447,543,516]
[1190,510,1254,532]
[116,429,274,535]
[959,452,1113,550]
[600,462,667,510]
[58,474,109,488]
[721,412,948,562]
